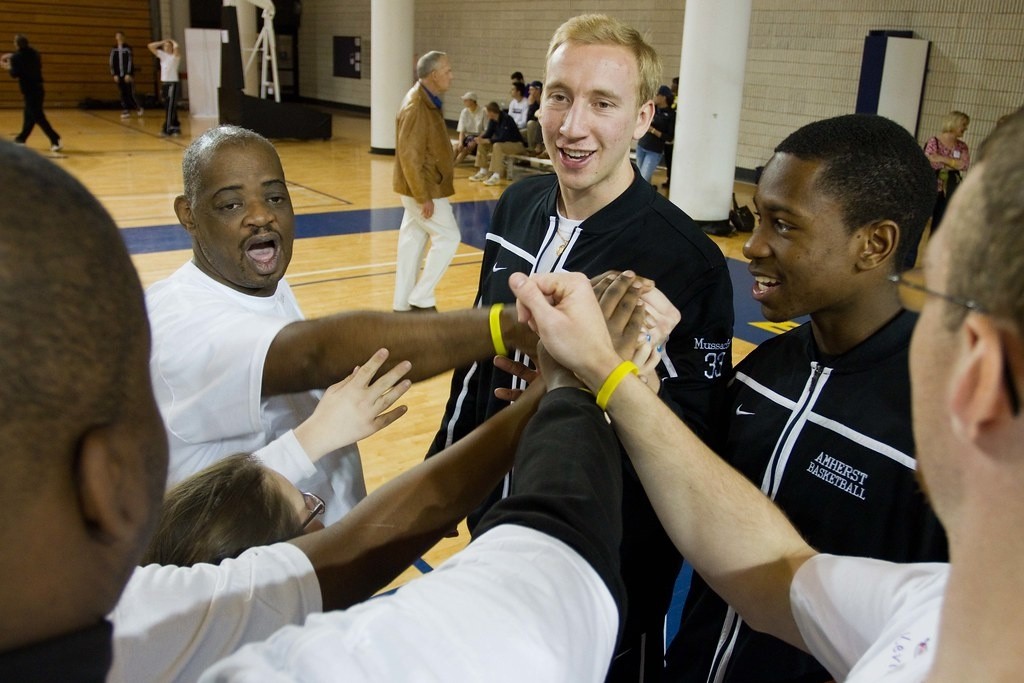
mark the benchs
[451,137,637,175]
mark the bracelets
[595,360,639,410]
[490,303,508,355]
[651,128,655,133]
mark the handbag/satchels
[730,192,755,232]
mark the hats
[659,85,672,96]
[528,80,543,88]
[460,92,478,103]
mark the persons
[0,106,1024,683]
[425,14,734,683]
[109,32,144,119]
[392,51,462,312]
[452,71,548,187]
[148,39,182,137]
[0,34,62,151]
[661,77,679,188]
[636,85,677,183]
[901,110,971,269]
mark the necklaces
[557,230,568,256]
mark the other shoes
[537,151,550,159]
[171,132,182,136]
[526,150,536,157]
[50,138,62,151]
[483,175,500,185]
[662,183,669,188]
[158,132,169,137]
[410,305,438,312]
[120,111,131,118]
[137,107,144,116]
[468,171,488,182]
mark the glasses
[289,489,326,538]
[888,267,1020,420]
